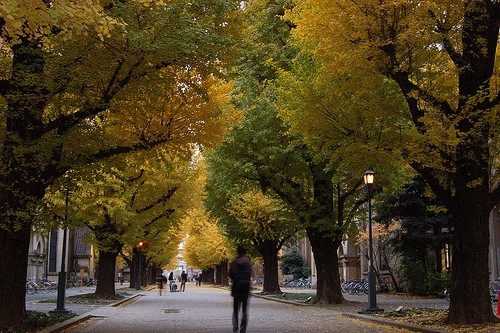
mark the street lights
[49,169,75,314]
[360,169,388,314]
[135,240,144,289]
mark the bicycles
[26,277,58,294]
[487,276,500,303]
[341,278,379,297]
[278,276,313,291]
[445,286,450,300]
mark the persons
[117,274,125,285]
[229,247,251,333]
[156,271,187,296]
[195,274,202,286]
